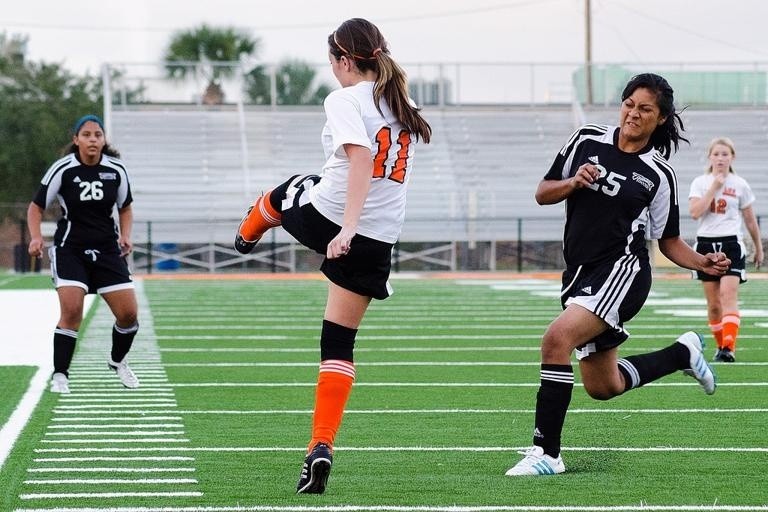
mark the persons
[688,136,764,362]
[504,72,731,476]
[234,17,432,494]
[27,114,139,394]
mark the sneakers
[107,360,140,389]
[712,349,735,362]
[50,373,70,394]
[235,206,262,254]
[296,442,333,495]
[676,330,717,395]
[505,445,565,477]
